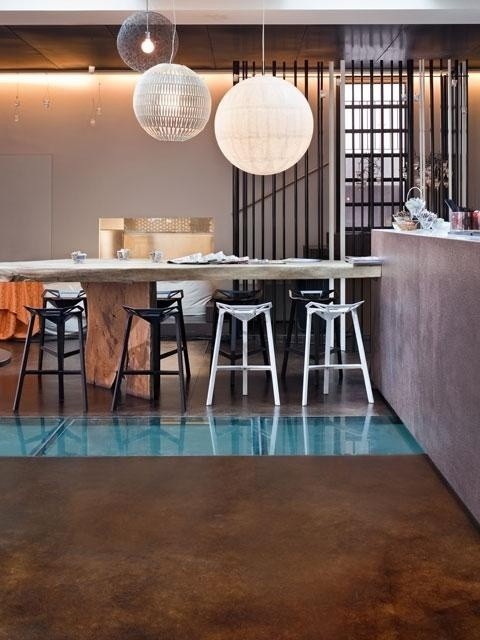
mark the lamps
[115,0,313,176]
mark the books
[346,256,382,266]
[167,251,249,264]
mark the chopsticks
[444,199,459,212]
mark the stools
[156,289,190,382]
[210,289,269,376]
[280,287,344,384]
[205,302,280,405]
[13,305,88,414]
[41,287,88,378]
[109,304,187,413]
[302,301,374,406]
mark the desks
[0,258,382,402]
